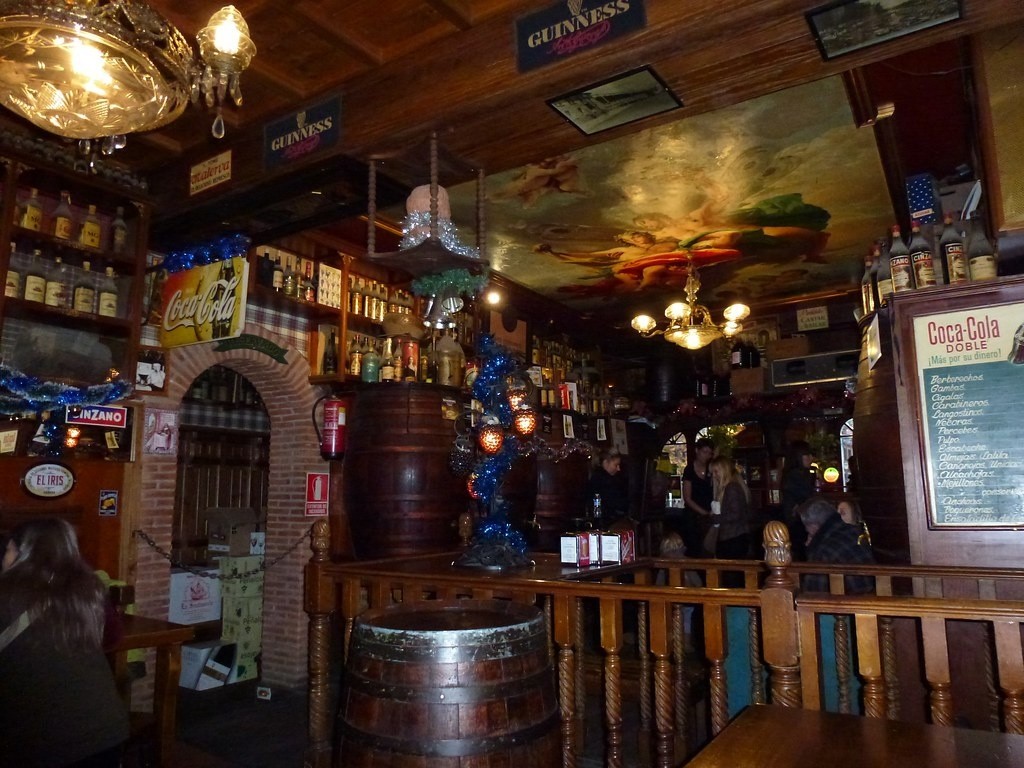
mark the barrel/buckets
[332,599,565,768]
[502,407,588,555]
[851,318,909,564]
[595,422,655,535]
[348,381,469,561]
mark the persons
[780,441,818,513]
[683,437,754,558]
[800,496,889,595]
[585,446,623,528]
[0,515,133,768]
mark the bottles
[107,207,127,253]
[861,209,997,313]
[745,336,760,367]
[257,249,612,417]
[49,190,72,240]
[72,261,95,314]
[0,128,149,195]
[97,267,118,317]
[23,249,46,303]
[708,376,720,398]
[75,206,100,248]
[212,258,235,339]
[4,242,20,298]
[45,257,65,307]
[696,373,708,399]
[732,332,746,368]
[18,187,42,231]
